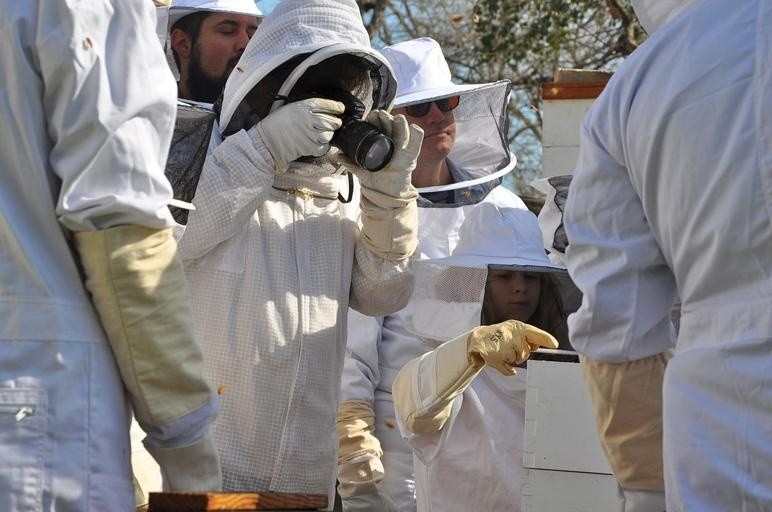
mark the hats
[415,185,568,274]
[169,0,265,19]
[381,37,510,109]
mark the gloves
[329,109,426,199]
[466,321,559,377]
[336,453,397,512]
[254,96,345,174]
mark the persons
[1,1,567,511]
[562,0,771,511]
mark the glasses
[405,95,461,118]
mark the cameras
[286,84,394,172]
